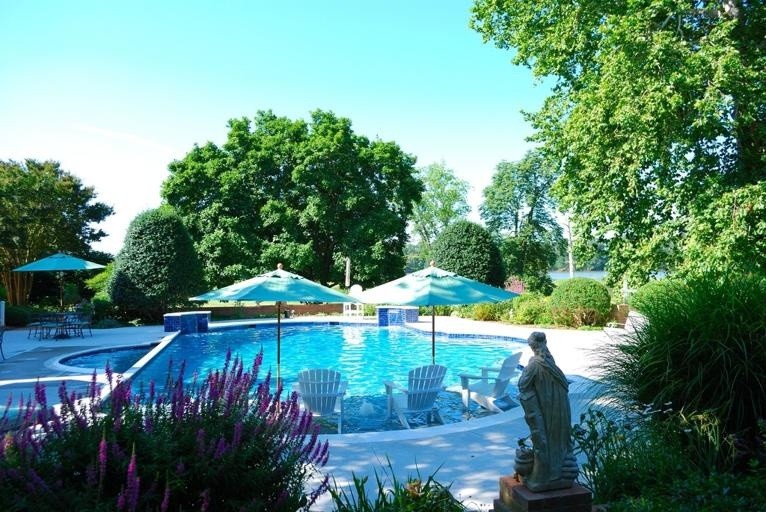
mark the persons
[518,332,579,494]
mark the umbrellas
[189,263,360,411]
[14,253,106,314]
[355,261,521,366]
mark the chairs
[445,351,523,421]
[22,304,93,342]
[370,366,449,432]
[289,371,350,435]
[343,284,364,316]
